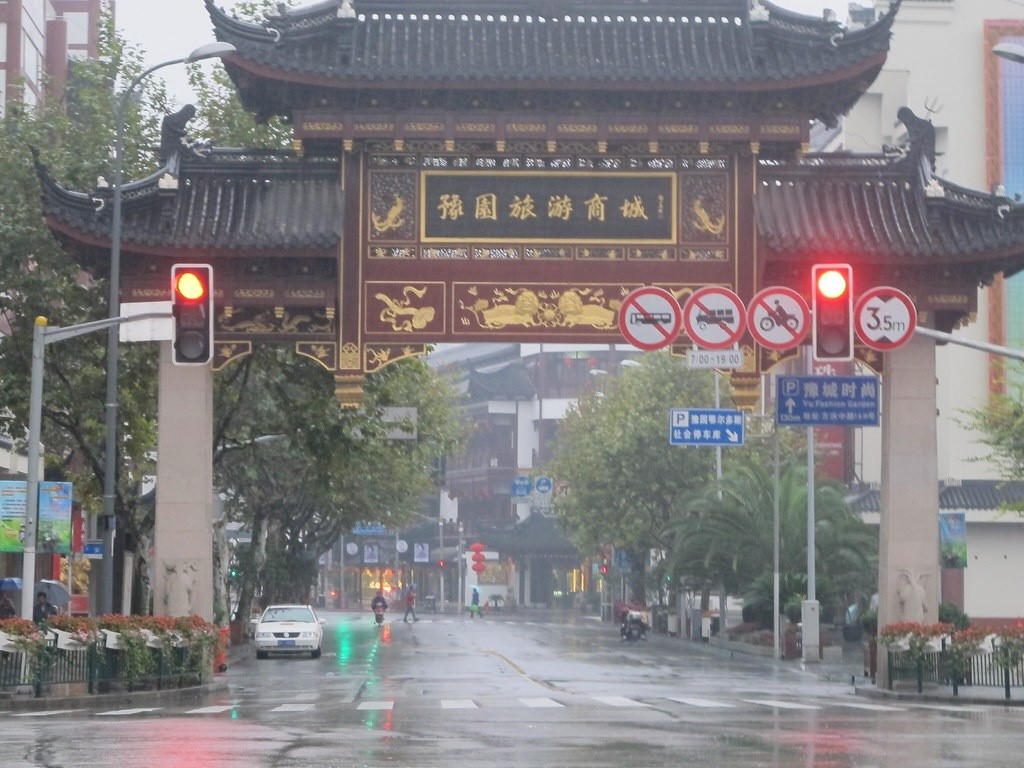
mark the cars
[251,603,327,659]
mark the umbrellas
[469,585,481,593]
[0,577,24,592]
[34,578,71,608]
[845,602,862,623]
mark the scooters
[373,601,386,627]
[619,610,643,644]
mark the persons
[403,586,422,623]
[372,590,388,617]
[866,589,878,622]
[415,543,427,558]
[0,591,16,622]
[620,600,648,643]
[33,592,58,624]
[469,588,484,618]
[365,546,377,559]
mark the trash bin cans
[667,613,681,637]
[700,616,720,641]
[212,627,231,673]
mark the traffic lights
[171,263,215,366]
[812,261,854,362]
[439,559,445,572]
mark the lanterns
[470,542,486,571]
